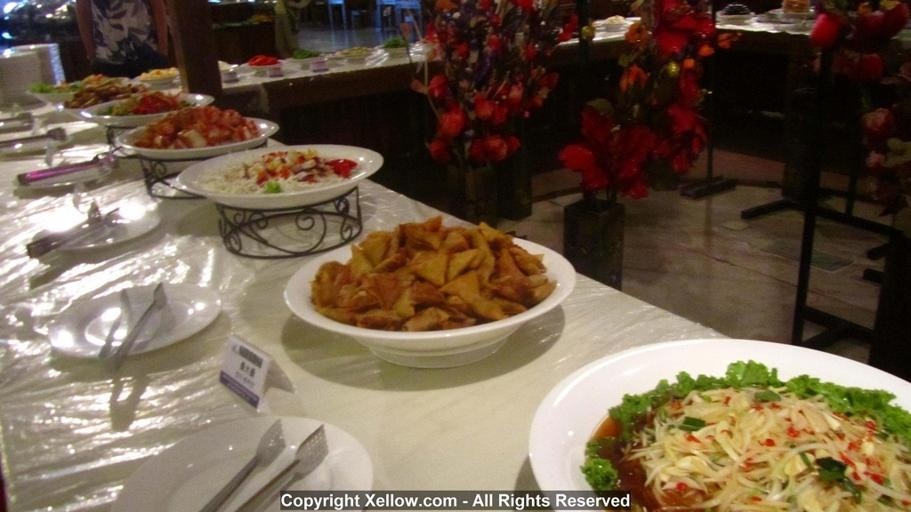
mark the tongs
[198,418,329,512]
[97,282,167,370]
[24,200,124,259]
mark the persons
[381,4,393,19]
[73,0,174,81]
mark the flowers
[809,0,910,218]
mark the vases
[561,197,623,294]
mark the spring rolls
[309,212,558,336]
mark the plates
[0,111,114,190]
[175,144,384,217]
[602,21,627,32]
[34,205,162,253]
[46,281,223,360]
[25,76,278,159]
[137,42,413,87]
[717,10,755,25]
[0,43,66,110]
[768,9,814,24]
[111,415,374,512]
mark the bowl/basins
[283,228,579,368]
[525,336,911,511]
